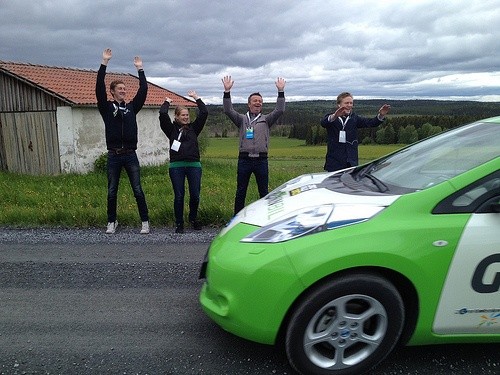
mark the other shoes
[191,221,204,230]
[175,223,185,234]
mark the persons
[95,48,150,234]
[159,90,208,234]
[222,75,287,226]
[320,92,391,172]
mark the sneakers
[139,221,151,234]
[106,220,118,234]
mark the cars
[199,116,500,375]
